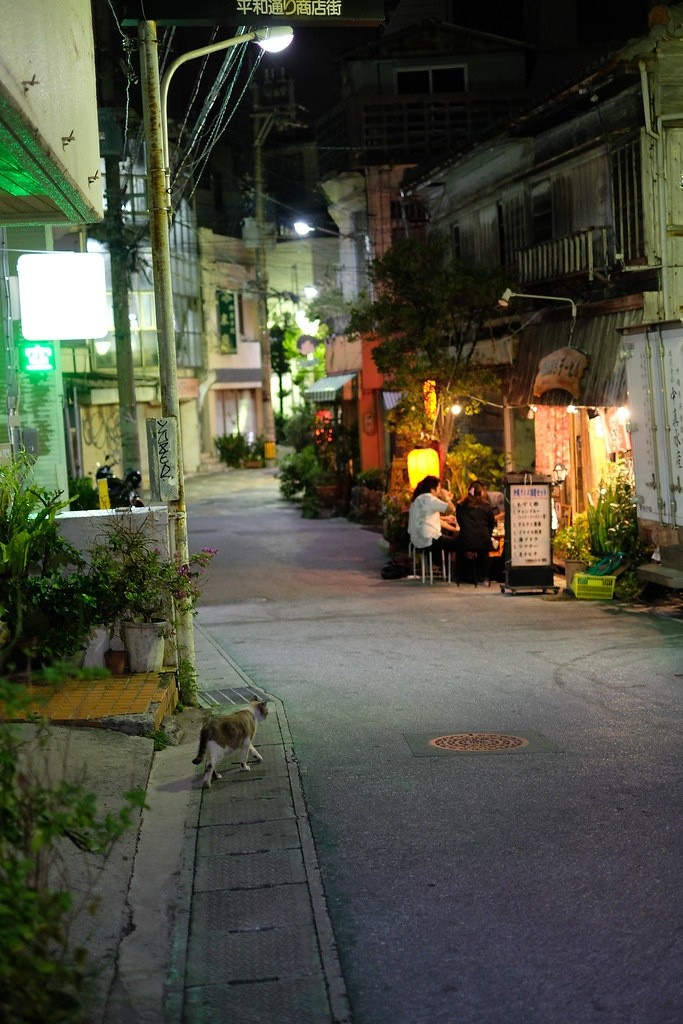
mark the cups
[440,488,454,502]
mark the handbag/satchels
[381,560,411,579]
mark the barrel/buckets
[104,648,128,676]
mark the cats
[191,693,269,790]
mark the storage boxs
[573,574,617,600]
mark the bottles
[497,513,505,535]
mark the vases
[121,617,169,673]
[105,652,127,675]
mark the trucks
[612,316,682,591]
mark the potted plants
[313,469,338,500]
[381,485,412,554]
[550,512,602,590]
[352,468,387,514]
[0,440,114,681]
[214,433,264,468]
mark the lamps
[498,288,577,347]
[554,464,568,490]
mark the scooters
[85,453,144,510]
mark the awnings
[302,372,360,404]
[381,392,408,411]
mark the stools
[408,542,491,588]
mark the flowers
[84,529,219,666]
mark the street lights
[136,23,297,712]
[293,222,379,336]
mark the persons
[456,482,506,580]
[407,476,464,566]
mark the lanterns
[407,445,440,489]
